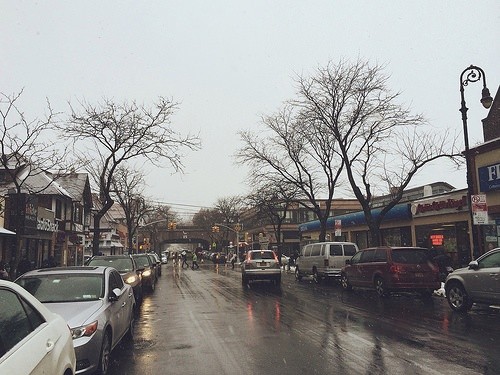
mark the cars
[130,253,162,290]
[340,246,443,299]
[444,248,500,310]
[13,267,134,375]
[241,248,281,283]
[277,254,290,266]
[84,253,141,307]
[0,280,76,375]
[195,247,227,263]
[294,240,358,284]
[161,253,167,263]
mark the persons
[47,256,57,268]
[181,251,189,268]
[289,250,300,266]
[191,252,199,269]
[230,253,235,269]
[18,255,32,275]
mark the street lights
[460,62,493,268]
[215,222,242,265]
